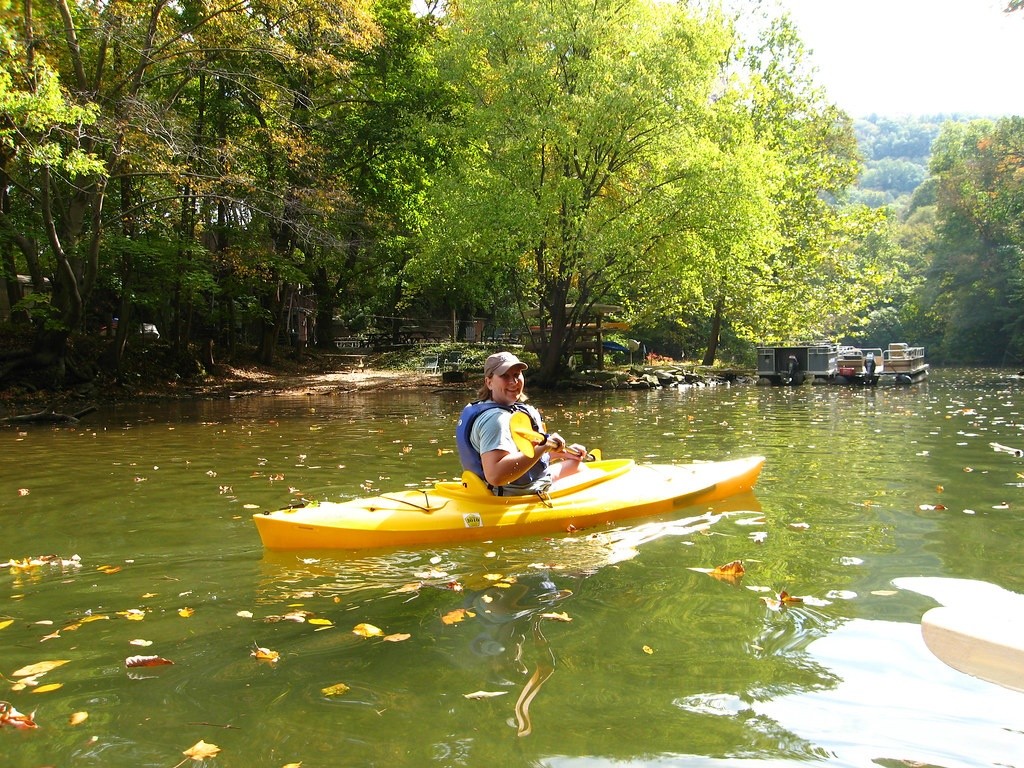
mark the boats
[829,343,930,386]
[522,339,630,358]
[514,321,628,334]
[516,303,625,314]
[253,455,766,554]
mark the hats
[484,352,528,377]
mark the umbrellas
[603,342,628,352]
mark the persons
[456,351,588,496]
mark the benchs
[319,363,372,369]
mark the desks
[318,354,369,374]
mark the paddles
[509,411,634,471]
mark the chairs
[481,324,523,343]
[444,351,470,372]
[416,353,439,375]
